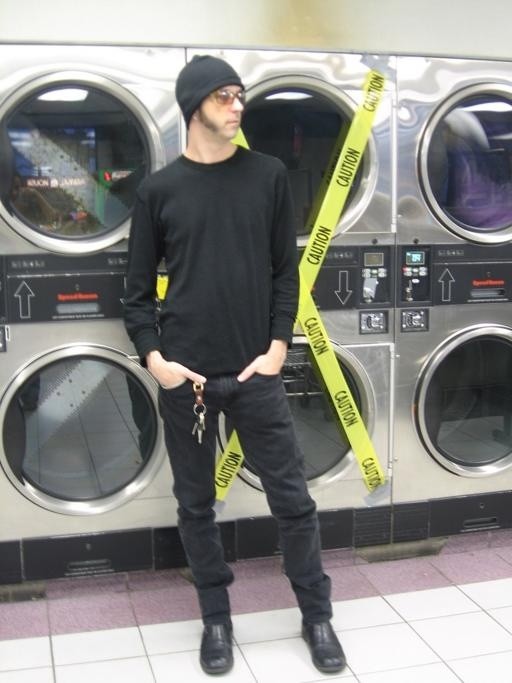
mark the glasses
[214,89,245,104]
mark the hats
[176,54,244,128]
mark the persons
[120,55,347,675]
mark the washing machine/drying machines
[0,41,181,571]
[184,41,392,561]
[393,45,510,540]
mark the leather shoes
[301,619,345,671]
[200,622,233,675]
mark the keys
[190,381,207,446]
